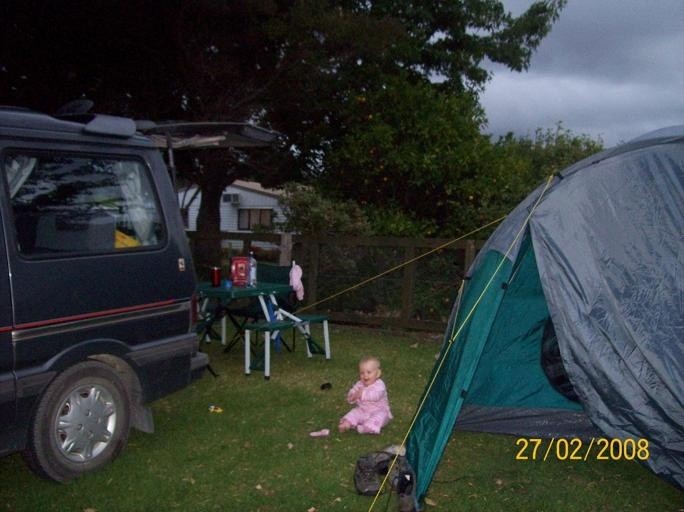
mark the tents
[395,125,683,512]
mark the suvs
[0,117,284,483]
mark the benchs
[243,314,331,380]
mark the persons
[338,356,393,435]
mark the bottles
[211,267,222,288]
[248,251,257,288]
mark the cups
[210,266,222,287]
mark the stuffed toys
[290,260,304,301]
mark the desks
[200,280,293,357]
